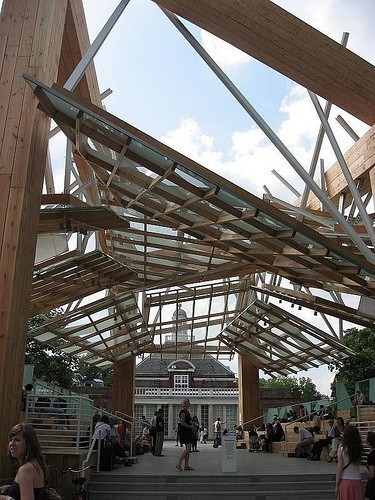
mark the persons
[0,421,45,500]
[335,426,366,500]
[21,384,366,471]
[363,430,375,500]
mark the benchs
[18,412,96,469]
[237,408,375,466]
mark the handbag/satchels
[45,486,62,500]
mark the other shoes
[158,454,165,457]
[184,467,194,470]
[335,460,338,463]
[125,464,132,467]
[327,460,332,463]
[307,457,313,460]
[176,466,183,471]
[311,458,320,461]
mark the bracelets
[9,496,13,500]
[8,485,10,488]
